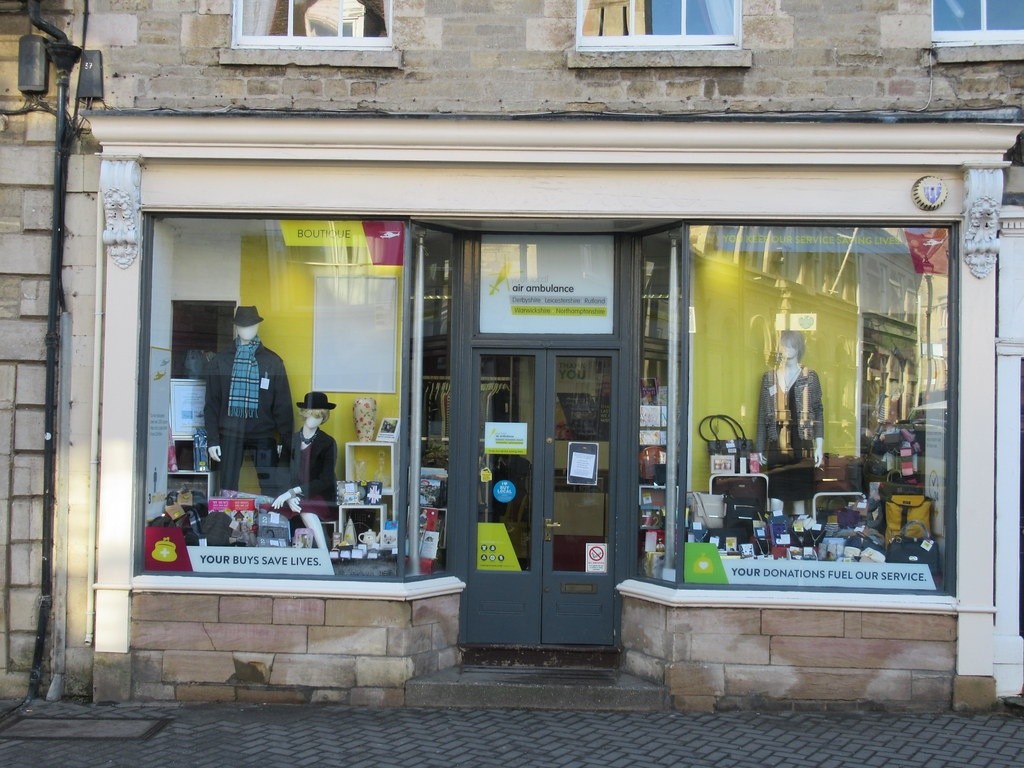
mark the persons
[270,391,339,567]
[201,305,293,498]
[756,330,826,515]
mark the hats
[296,391,337,410]
[230,306,265,326]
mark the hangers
[425,374,510,398]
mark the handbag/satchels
[699,415,755,474]
[879,468,943,586]
[691,477,766,528]
[814,452,863,494]
[639,446,669,480]
